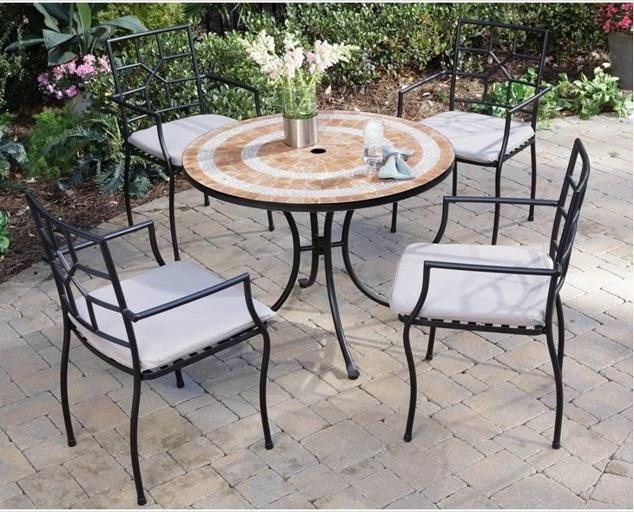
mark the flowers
[240,28,359,82]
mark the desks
[181,109,456,379]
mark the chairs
[102,25,274,263]
[26,193,276,507]
[391,18,550,245]
[390,137,590,444]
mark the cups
[282,112,319,149]
[362,118,385,167]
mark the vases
[282,107,321,149]
